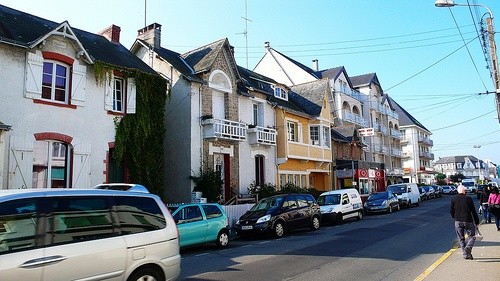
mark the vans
[316,188,363,225]
[235,193,322,239]
[462,178,499,199]
[0,183,181,281]
[387,182,421,208]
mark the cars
[363,191,400,216]
[167,203,232,251]
[418,184,457,201]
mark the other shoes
[484,220,491,223]
[497,226,500,231]
[463,246,473,260]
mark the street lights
[434,0,500,124]
[473,144,482,180]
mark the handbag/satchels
[478,205,483,216]
[488,204,494,214]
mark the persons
[450,185,480,260]
[480,184,500,231]
